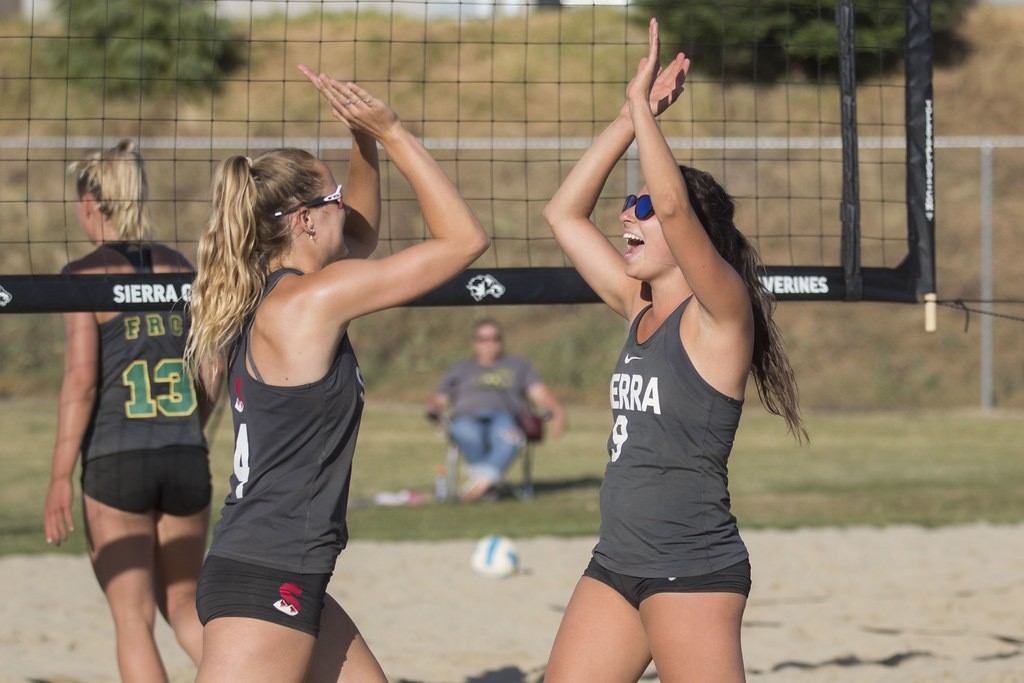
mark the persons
[542,17,811,683]
[186,65,492,683]
[430,315,569,502]
[44,138,225,683]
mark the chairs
[426,399,552,499]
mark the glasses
[622,194,654,220]
[275,184,342,216]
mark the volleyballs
[474,533,519,578]
